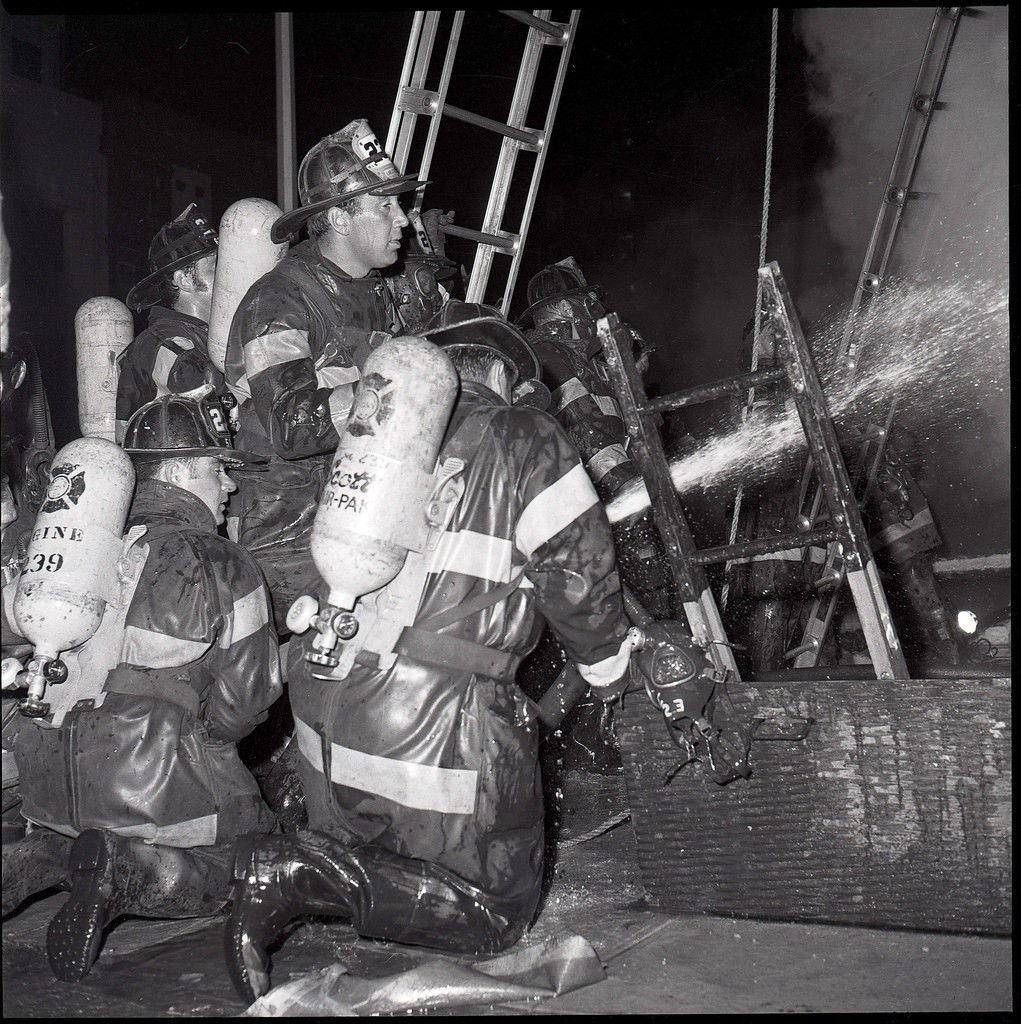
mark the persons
[223,114,449,637]
[737,302,961,668]
[111,209,242,544]
[1,397,283,919]
[520,257,639,500]
[47,300,634,1008]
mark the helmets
[121,394,271,472]
[416,297,553,412]
[125,216,218,315]
[514,263,602,330]
[270,133,434,245]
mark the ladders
[380,4,587,320]
[591,264,911,684]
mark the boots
[222,831,423,1004]
[0,829,233,983]
